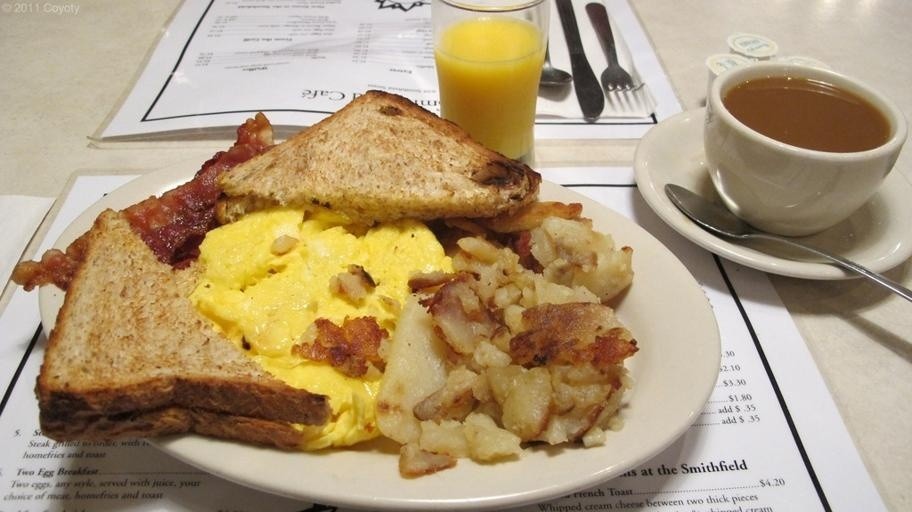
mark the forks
[584,1,642,114]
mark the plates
[38,154,722,510]
[633,106,912,281]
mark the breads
[214,91,580,232]
[34,207,330,450]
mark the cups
[704,61,908,238]
[432,1,552,162]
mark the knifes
[556,0,604,121]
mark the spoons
[663,182,912,304]
[526,6,573,89]
